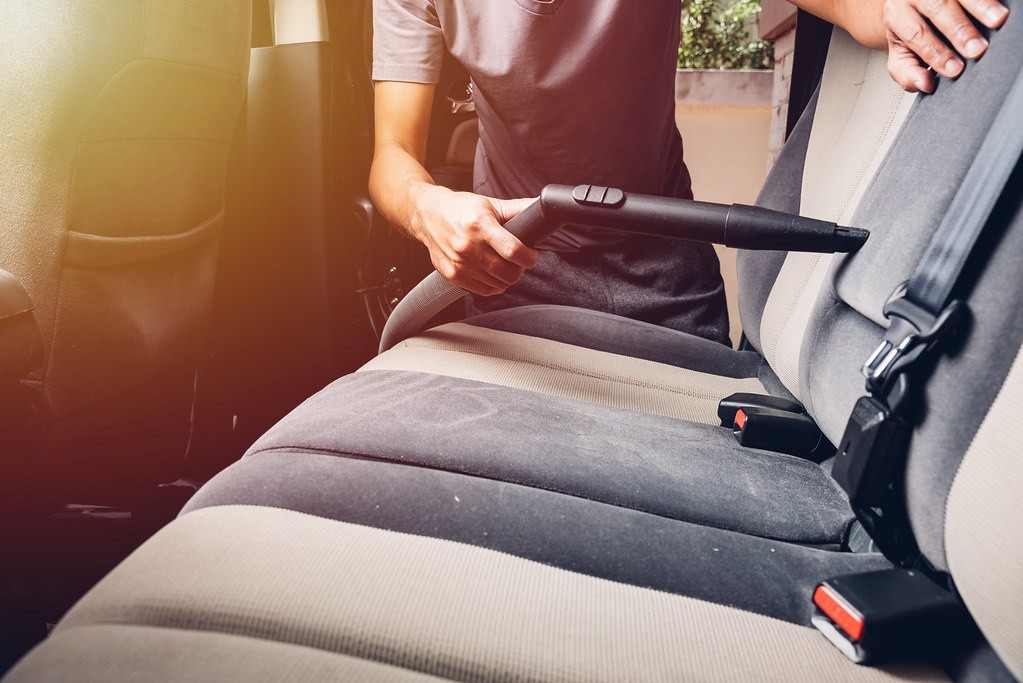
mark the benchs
[2,0,1022,682]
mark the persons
[368,0,1011,350]
